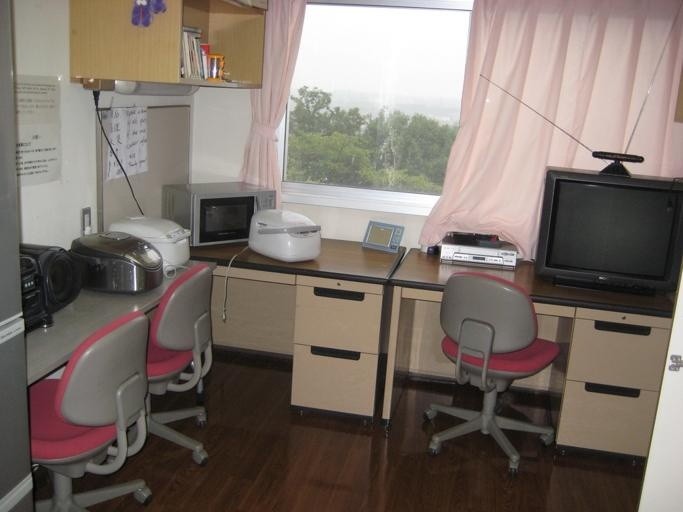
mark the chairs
[27,311,153,511]
[422,271,561,478]
[108,262,213,467]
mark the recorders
[19,243,84,334]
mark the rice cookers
[70,209,321,295]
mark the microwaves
[162,181,277,247]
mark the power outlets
[82,208,91,236]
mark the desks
[27,261,216,386]
[187,239,406,436]
[382,248,674,474]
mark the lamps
[115,81,199,97]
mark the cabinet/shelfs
[70,1,269,89]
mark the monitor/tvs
[533,151,683,298]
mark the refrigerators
[0,1,34,511]
